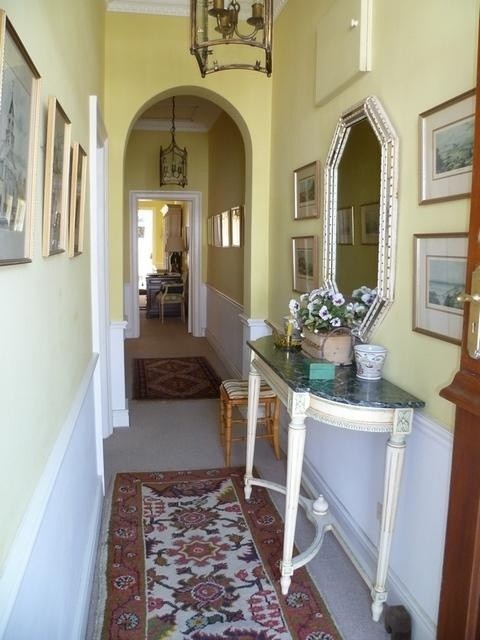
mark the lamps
[160,94,188,188]
[164,234,186,273]
[189,0,273,78]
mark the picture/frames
[359,202,380,245]
[231,205,241,248]
[293,159,320,220]
[214,213,221,248]
[411,232,469,346]
[222,210,230,248]
[292,234,318,292]
[337,206,354,245]
[417,87,477,207]
[0,10,40,267]
[208,216,213,246]
[70,142,88,258]
[42,96,73,257]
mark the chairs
[156,271,187,325]
[220,330,281,468]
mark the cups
[354,345,389,380]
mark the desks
[244,335,426,622]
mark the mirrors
[319,95,400,344]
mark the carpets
[132,356,224,400]
[99,463,347,640]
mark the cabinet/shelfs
[146,277,183,319]
[159,204,181,270]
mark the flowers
[345,286,378,326]
[289,286,353,334]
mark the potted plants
[300,325,355,366]
